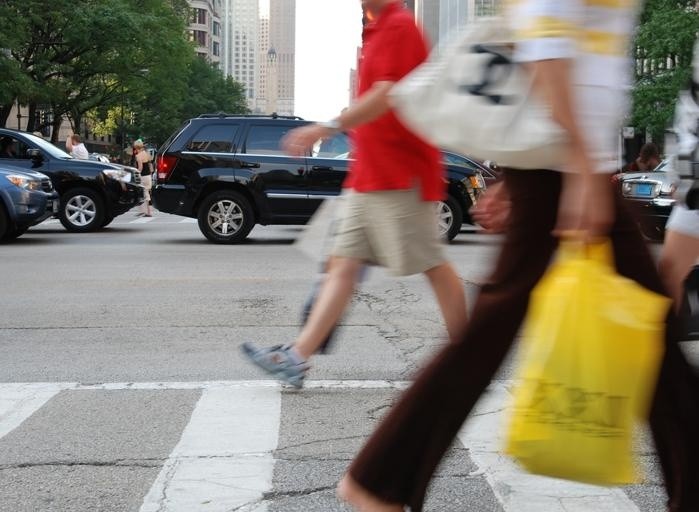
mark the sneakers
[135,212,152,218]
[241,340,307,392]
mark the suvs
[619,154,675,240]
[150,110,502,245]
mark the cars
[0,126,145,240]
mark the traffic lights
[133,113,139,125]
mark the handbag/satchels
[387,14,589,170]
[505,232,676,492]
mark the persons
[653,41,699,319]
[132,139,154,218]
[238,1,472,388]
[66,134,90,160]
[329,0,699,510]
[33,129,43,138]
[3,136,18,157]
[635,142,660,172]
